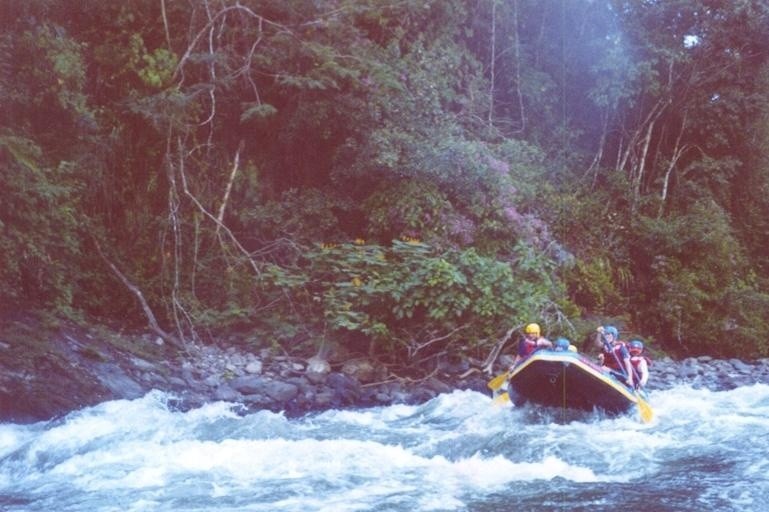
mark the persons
[506,323,552,375]
[553,333,580,353]
[598,339,649,398]
[596,323,634,390]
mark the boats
[508,350,640,426]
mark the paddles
[491,393,509,408]
[487,346,543,391]
[597,326,652,423]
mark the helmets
[602,327,618,341]
[525,323,541,335]
[629,340,643,355]
[555,339,577,352]
